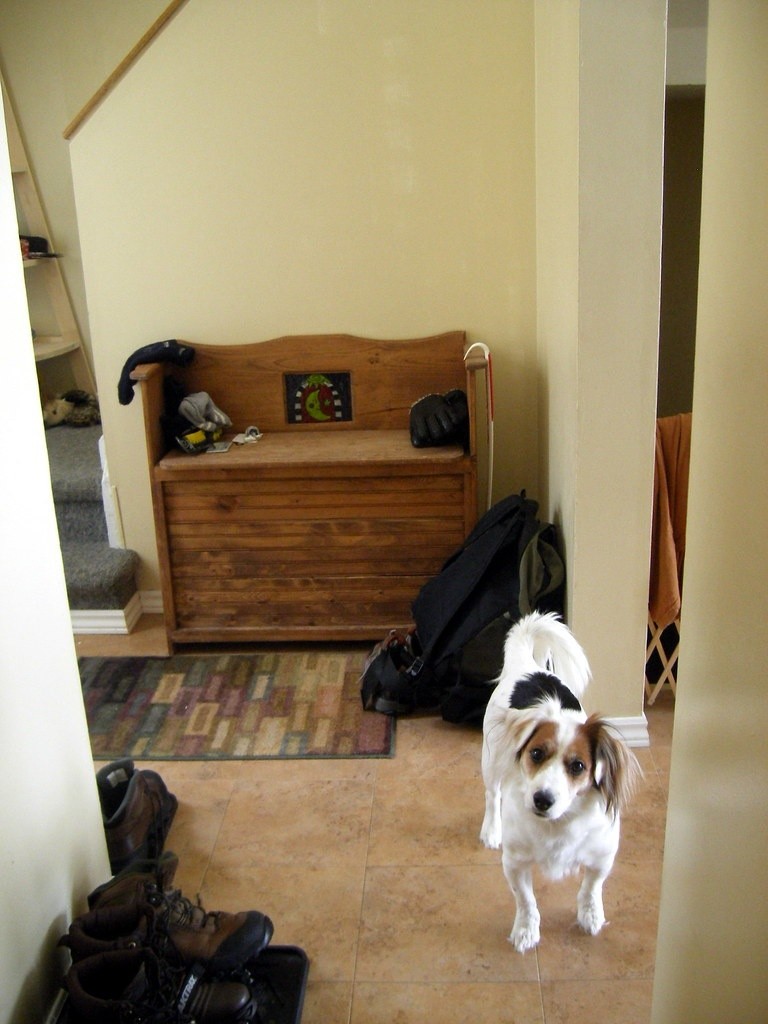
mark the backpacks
[360,490,545,726]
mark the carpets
[78,654,396,760]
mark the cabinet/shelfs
[0,64,100,432]
[126,330,489,659]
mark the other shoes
[59,904,253,1024]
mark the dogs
[478,609,640,956]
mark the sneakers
[96,758,168,874]
[86,872,273,975]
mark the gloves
[409,388,473,448]
